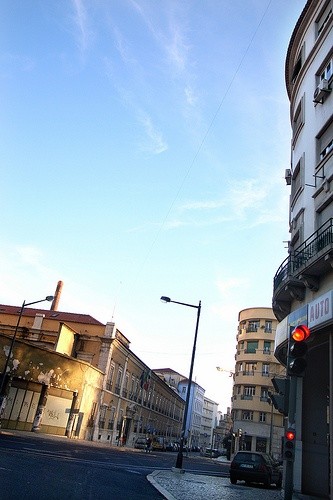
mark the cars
[205,448,228,458]
[134,436,201,452]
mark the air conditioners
[284,168,291,185]
[312,79,328,102]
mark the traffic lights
[286,324,311,378]
[238,428,243,438]
[283,428,297,461]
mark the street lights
[0,295,55,394]
[160,296,202,474]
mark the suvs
[228,450,283,490]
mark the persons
[146,438,151,453]
